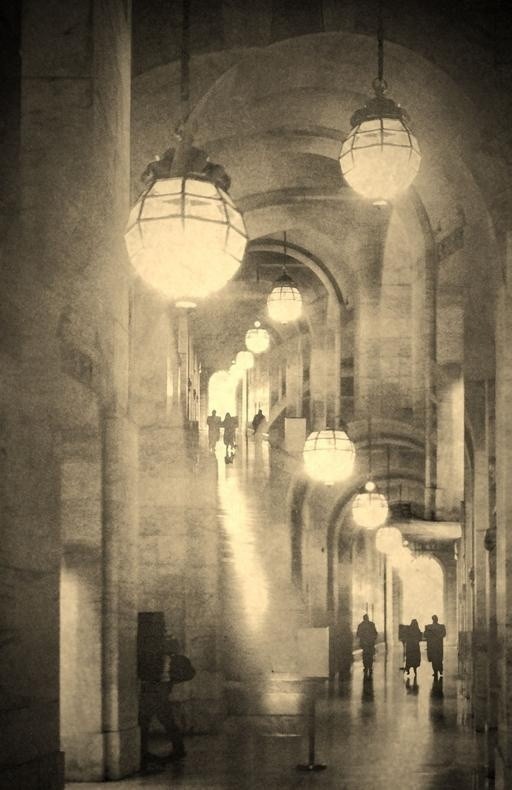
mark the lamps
[121,0,424,572]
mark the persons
[250,409,267,446]
[424,614,447,676]
[135,611,183,763]
[146,617,187,766]
[221,412,237,450]
[361,672,378,736]
[404,677,420,719]
[356,613,378,672]
[206,409,222,452]
[402,618,422,676]
[253,446,266,489]
[223,451,236,483]
[427,676,448,738]
